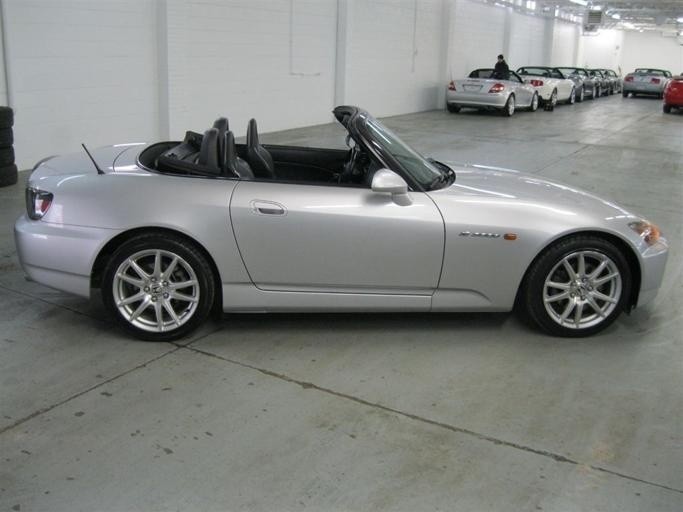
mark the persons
[494,55,509,82]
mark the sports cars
[446,68,539,117]
[515,66,682,114]
[14,105,669,343]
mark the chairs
[246,118,275,181]
[222,129,253,179]
